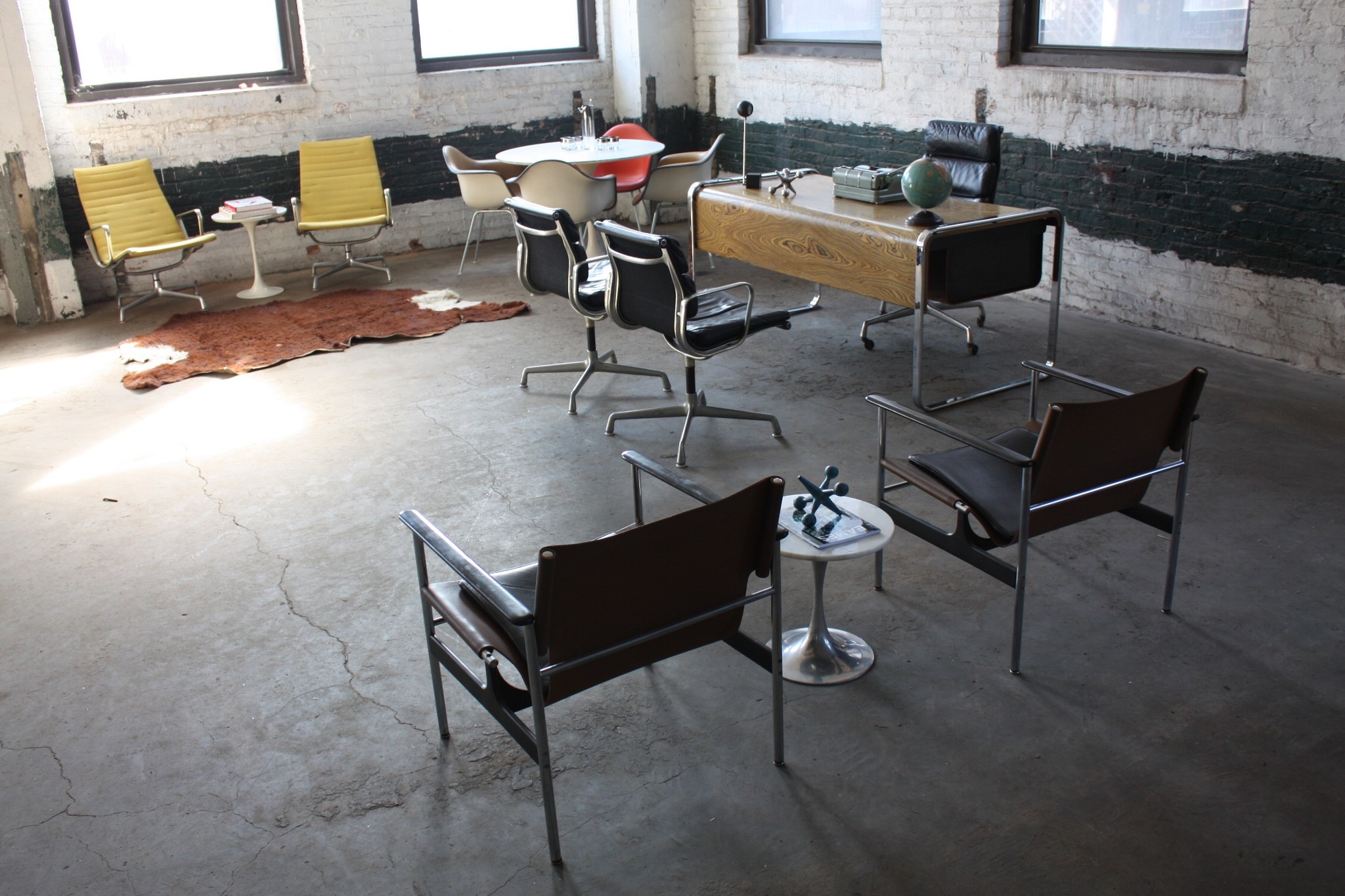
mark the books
[777,503,880,550]
[218,196,278,219]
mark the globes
[902,152,953,226]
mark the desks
[495,135,667,265]
[212,206,287,299]
[772,494,896,685]
[687,168,1067,413]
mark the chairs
[73,158,217,324]
[503,196,673,415]
[291,135,395,291]
[594,123,658,233]
[506,158,620,227]
[861,120,1004,354]
[632,133,725,275]
[593,219,791,467]
[866,360,1208,673]
[442,146,524,275]
[399,450,785,866]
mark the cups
[559,135,619,151]
